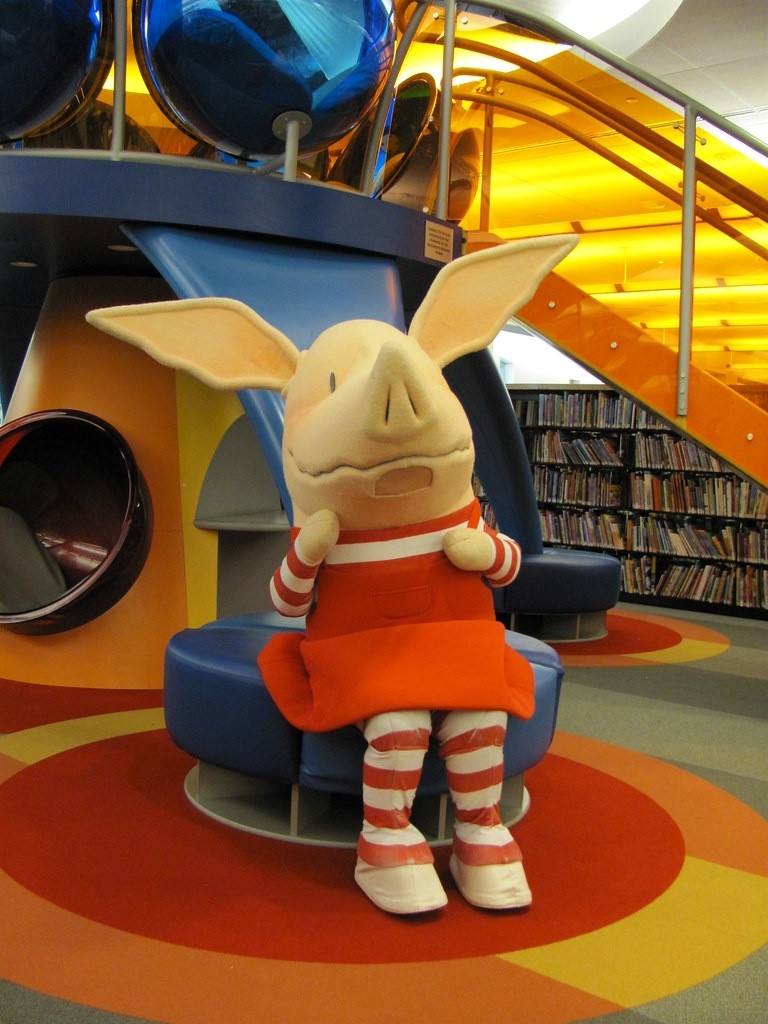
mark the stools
[510,548,621,649]
[160,605,567,859]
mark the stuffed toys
[84,235,581,919]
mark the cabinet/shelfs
[475,382,768,622]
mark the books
[500,375,768,612]
